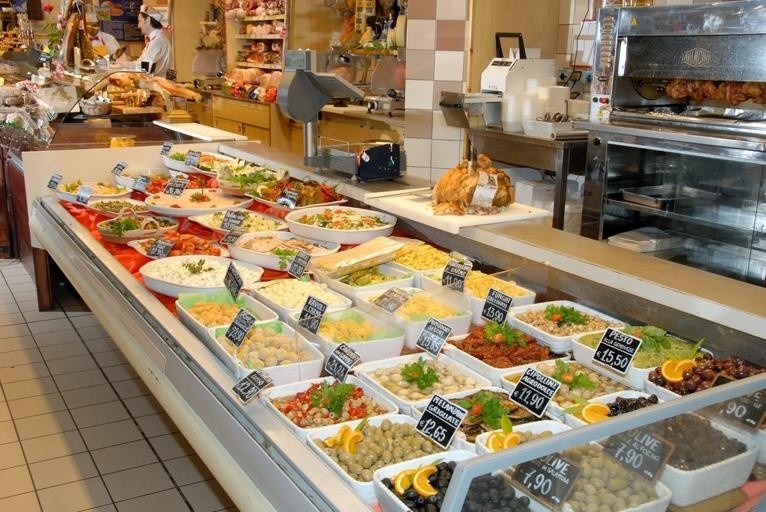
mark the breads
[0,86,54,149]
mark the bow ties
[89,36,98,41]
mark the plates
[97,207,182,243]
[127,240,229,260]
[356,352,492,413]
[174,293,280,344]
[80,198,146,215]
[213,170,288,197]
[371,451,527,511]
[474,420,673,512]
[565,390,757,505]
[52,181,133,203]
[206,322,325,389]
[573,329,715,389]
[311,415,459,503]
[283,207,398,243]
[186,213,288,235]
[411,387,557,449]
[286,309,406,371]
[420,271,537,326]
[444,332,573,386]
[136,255,265,297]
[229,233,341,272]
[355,289,474,350]
[243,191,350,213]
[504,303,631,353]
[643,365,764,466]
[144,188,249,217]
[502,364,635,414]
[258,375,400,442]
[388,256,474,285]
[318,265,412,304]
[251,281,351,323]
[111,144,236,196]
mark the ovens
[610,0,766,139]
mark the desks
[462,129,589,230]
[0,41,191,313]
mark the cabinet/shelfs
[29,164,766,510]
[207,100,290,156]
[317,103,405,157]
[173,82,213,126]
[224,1,343,102]
[170,0,225,97]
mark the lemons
[394,466,440,497]
[581,401,611,421]
[660,359,701,382]
[486,430,519,452]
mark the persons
[108,3,170,69]
[84,23,126,63]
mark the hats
[140,4,163,22]
[86,13,99,23]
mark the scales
[278,50,407,184]
